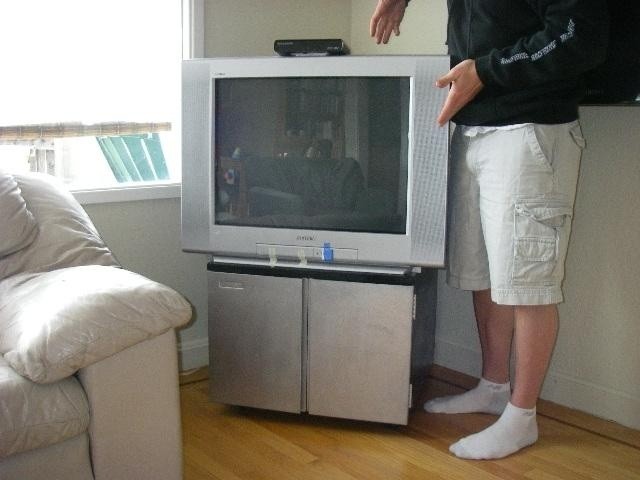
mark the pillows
[0,268,193,384]
[0,162,38,259]
[0,168,121,279]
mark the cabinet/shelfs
[205,253,438,426]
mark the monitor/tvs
[179,54,451,278]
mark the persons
[368,1,591,461]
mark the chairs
[0,167,184,480]
